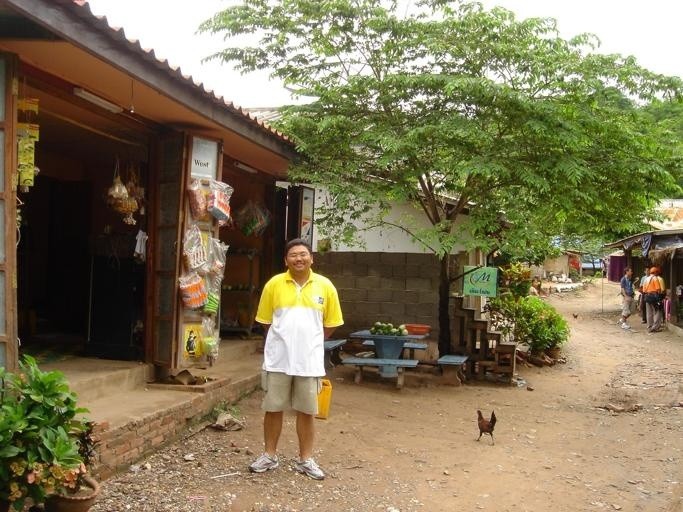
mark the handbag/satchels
[645,293,664,311]
[314,378,333,421]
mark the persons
[638,265,667,334]
[528,279,540,297]
[616,267,635,330]
[247,238,345,480]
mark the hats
[650,267,661,273]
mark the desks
[349,329,430,378]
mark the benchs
[323,339,472,391]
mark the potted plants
[43,420,100,511]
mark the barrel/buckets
[315,380,332,419]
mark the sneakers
[617,318,631,330]
[248,452,279,473]
[296,456,325,480]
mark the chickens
[476,410,497,446]
[572,313,578,319]
